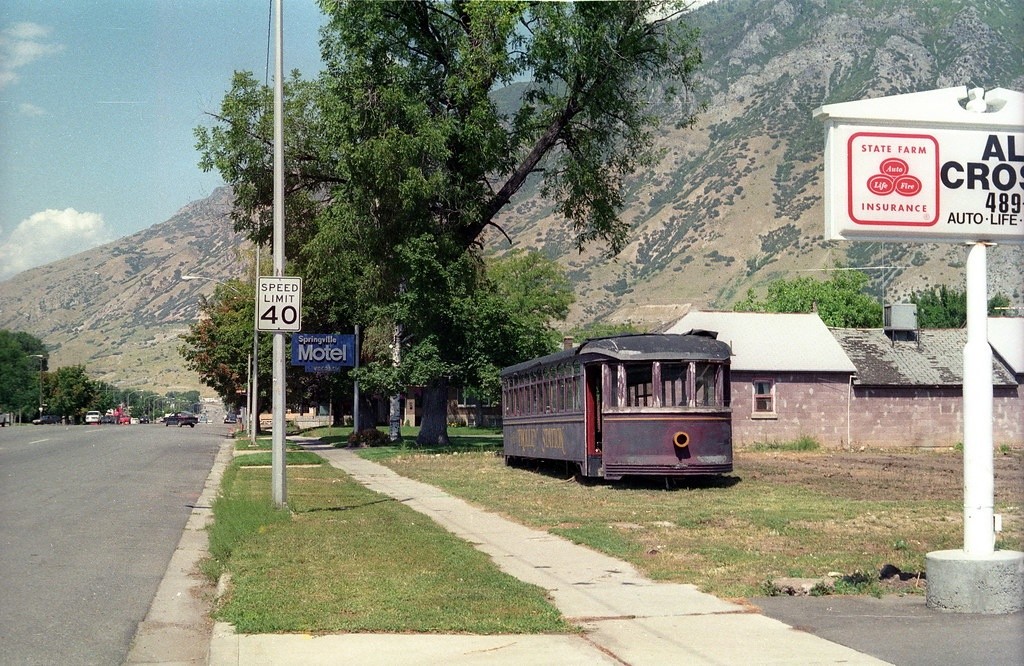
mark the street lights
[181,275,257,446]
[144,395,159,405]
[128,390,143,415]
[162,398,176,409]
[153,397,168,424]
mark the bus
[497,333,734,480]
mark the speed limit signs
[256,276,302,333]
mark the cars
[33,415,61,425]
[166,411,199,428]
[86,411,103,425]
[102,416,150,425]
